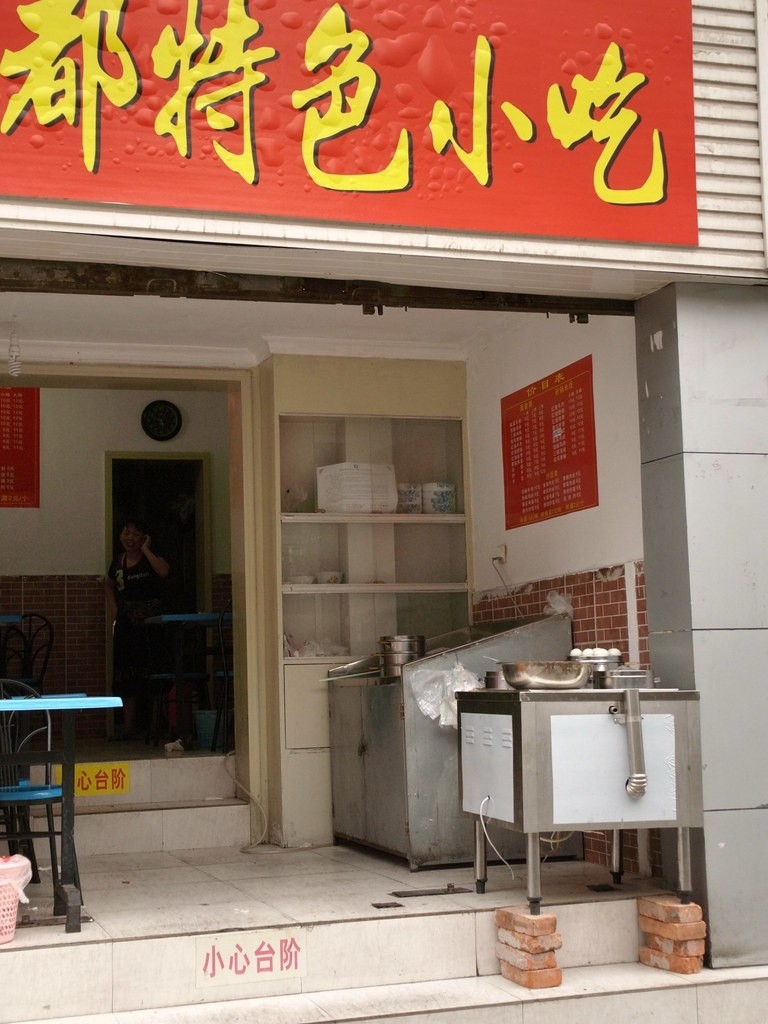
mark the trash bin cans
[0,854,33,943]
[194,709,226,747]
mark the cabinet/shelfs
[253,352,474,846]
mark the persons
[106,515,168,737]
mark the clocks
[140,400,182,441]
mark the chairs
[145,608,210,744]
[0,679,62,889]
[211,598,233,753]
[0,613,55,734]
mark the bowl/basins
[316,571,344,584]
[496,661,598,689]
[288,574,316,584]
[397,482,456,514]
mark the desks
[144,613,232,745]
[0,695,124,933]
